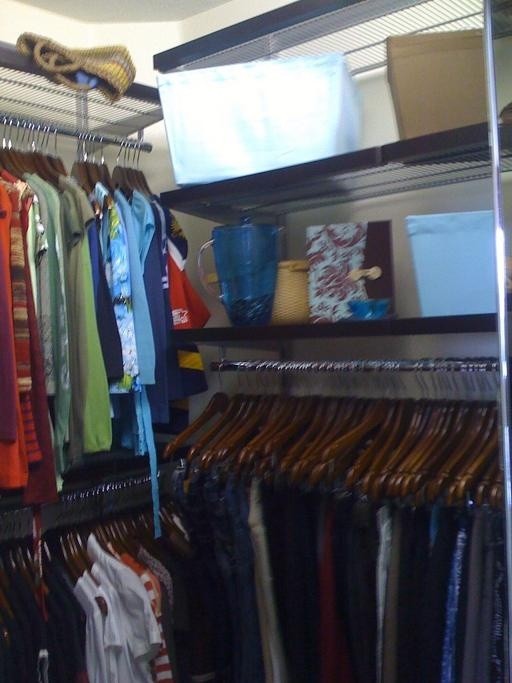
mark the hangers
[0,110,170,232]
[0,464,199,664]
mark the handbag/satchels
[16,32,135,102]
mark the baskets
[208,260,310,323]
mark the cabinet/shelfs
[151,1,510,348]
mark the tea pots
[196,216,287,326]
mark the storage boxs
[383,28,492,141]
